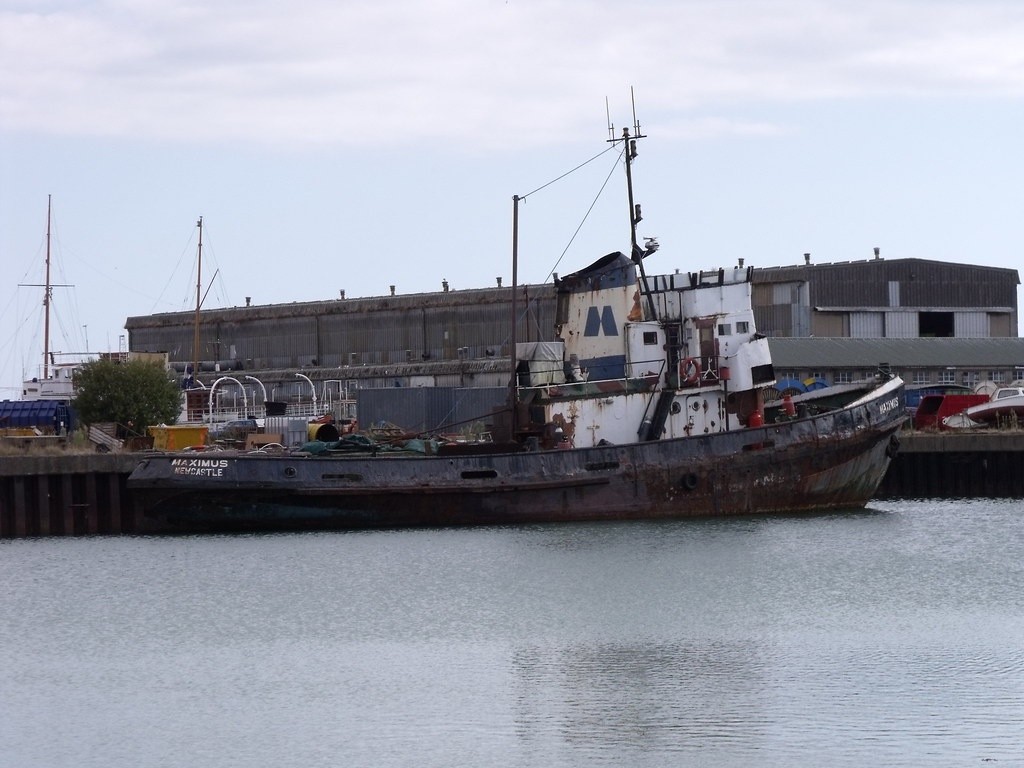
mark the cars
[991,387,1024,402]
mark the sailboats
[22,195,168,401]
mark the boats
[942,395,1024,428]
[122,85,910,533]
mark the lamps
[442,282,448,291]
[496,277,502,287]
[340,289,345,299]
[552,273,558,279]
[390,285,395,295]
[245,296,251,306]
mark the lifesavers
[681,357,701,383]
